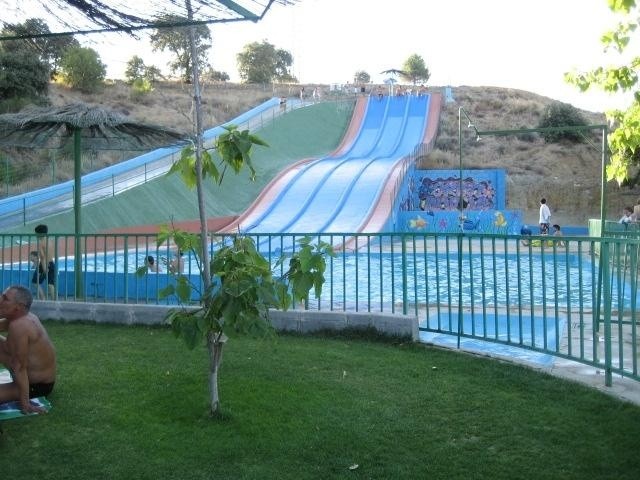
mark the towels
[0,365,52,420]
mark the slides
[200,93,430,254]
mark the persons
[279,78,426,113]
[617,207,637,224]
[421,198,426,211]
[144,256,162,272]
[0,286,56,416]
[31,225,57,300]
[539,198,551,234]
[522,225,564,247]
[30,251,40,270]
[170,250,184,273]
